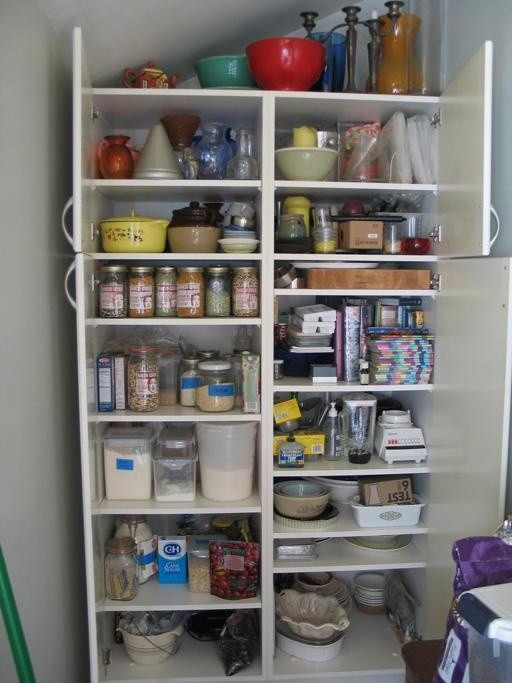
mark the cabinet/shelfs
[60,25,511,682]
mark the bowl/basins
[218,238,259,252]
[401,237,432,254]
[352,572,386,616]
[275,589,350,640]
[276,146,340,181]
[192,54,255,89]
[244,35,327,89]
[377,212,431,237]
[295,571,332,589]
[378,192,426,213]
[273,476,363,519]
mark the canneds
[209,517,240,539]
[105,516,154,601]
[126,345,255,412]
[97,263,259,318]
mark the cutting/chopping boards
[305,268,431,290]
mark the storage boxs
[454,582,511,682]
[152,453,200,503]
[102,426,153,501]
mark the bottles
[369,12,424,92]
[357,359,370,384]
[103,538,141,602]
[324,402,345,460]
[197,120,258,179]
[210,516,236,537]
[234,324,253,352]
[277,431,304,468]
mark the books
[331,297,435,386]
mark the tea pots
[122,60,179,89]
[95,131,136,179]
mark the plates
[312,537,335,545]
[274,266,308,288]
[219,229,257,238]
[291,261,379,269]
[344,534,414,553]
[292,579,351,611]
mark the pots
[98,205,170,253]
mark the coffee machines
[343,391,428,466]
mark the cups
[274,361,284,380]
[304,30,348,90]
[161,112,199,181]
[380,222,401,253]
[405,0,445,93]
[222,202,256,231]
[277,195,342,254]
